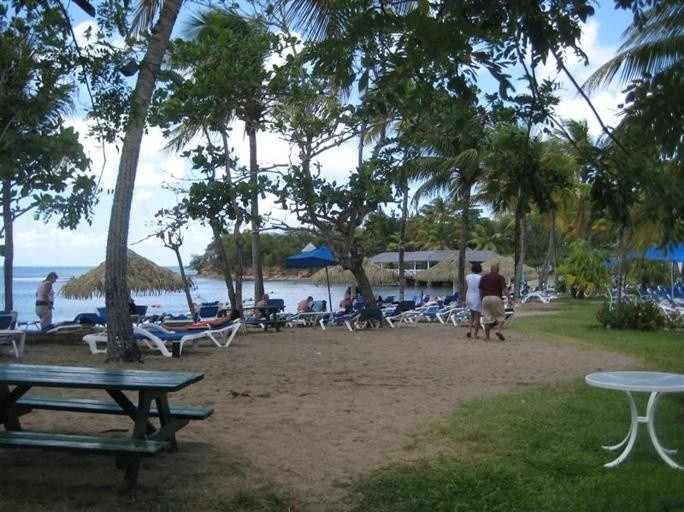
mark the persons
[297,296,313,327]
[311,300,326,326]
[36,272,57,334]
[254,293,270,320]
[162,310,240,331]
[463,263,483,339]
[478,264,512,342]
[339,292,356,313]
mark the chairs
[609,281,684,331]
[319,280,559,332]
[0,294,319,358]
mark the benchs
[1,392,216,440]
[2,431,172,489]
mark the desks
[583,370,684,477]
[0,358,202,480]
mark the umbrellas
[286,245,340,311]
[624,239,684,298]
